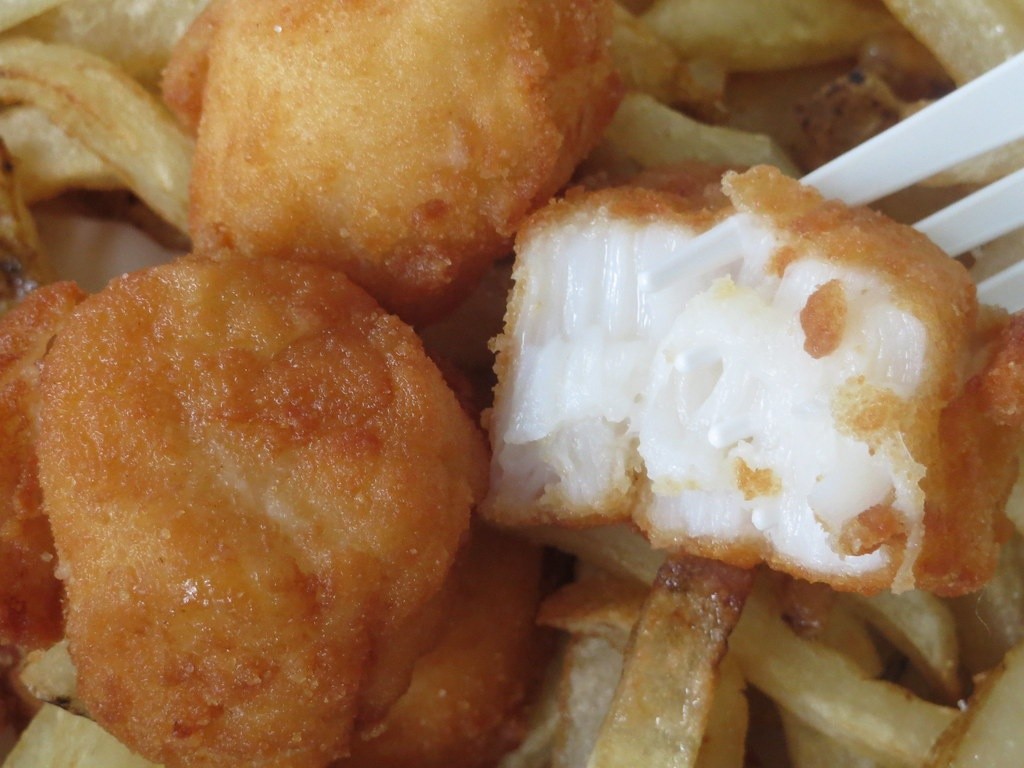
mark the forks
[798,52,1024,317]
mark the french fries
[0,0,1024,768]
